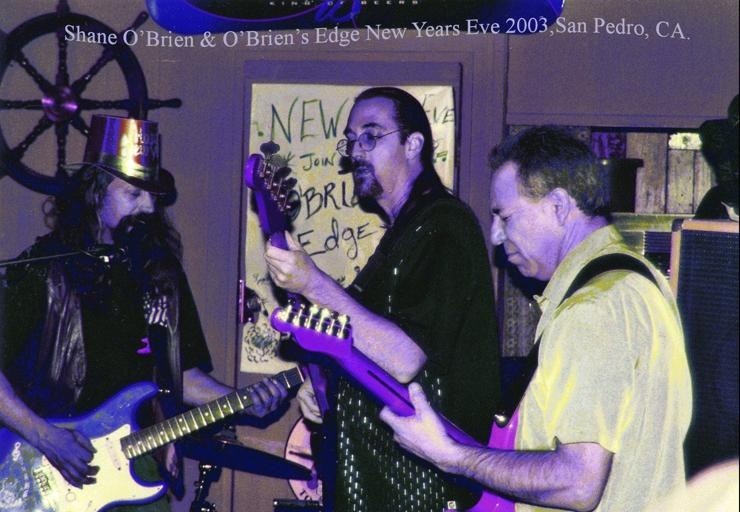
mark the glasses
[335,127,405,158]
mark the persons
[378,126,695,512]
[262,86,501,511]
[0,115,289,510]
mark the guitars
[243,141,337,512]
[270,296,518,512]
[0,363,306,512]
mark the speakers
[669,218,740,481]
[610,213,695,277]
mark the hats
[61,114,176,194]
